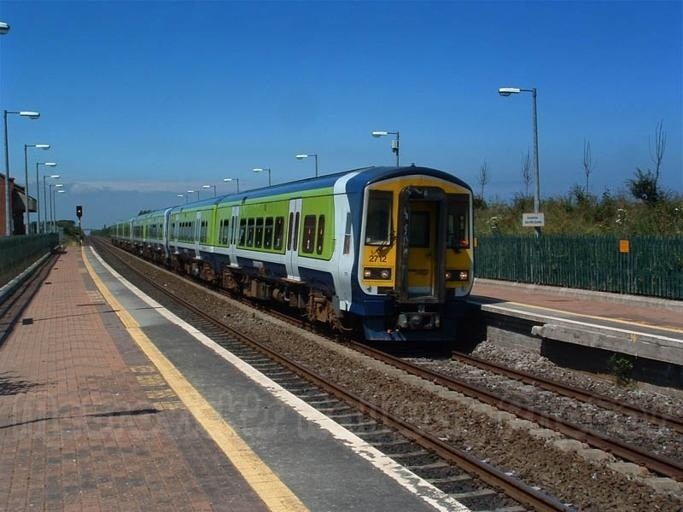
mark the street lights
[177,131,400,204]
[498,87,542,237]
[3,110,66,237]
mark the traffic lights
[76,205,82,219]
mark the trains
[109,164,475,345]
